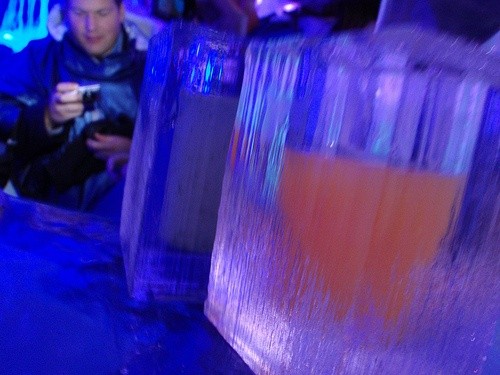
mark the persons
[0,0,150,220]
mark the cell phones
[79,84,102,103]
